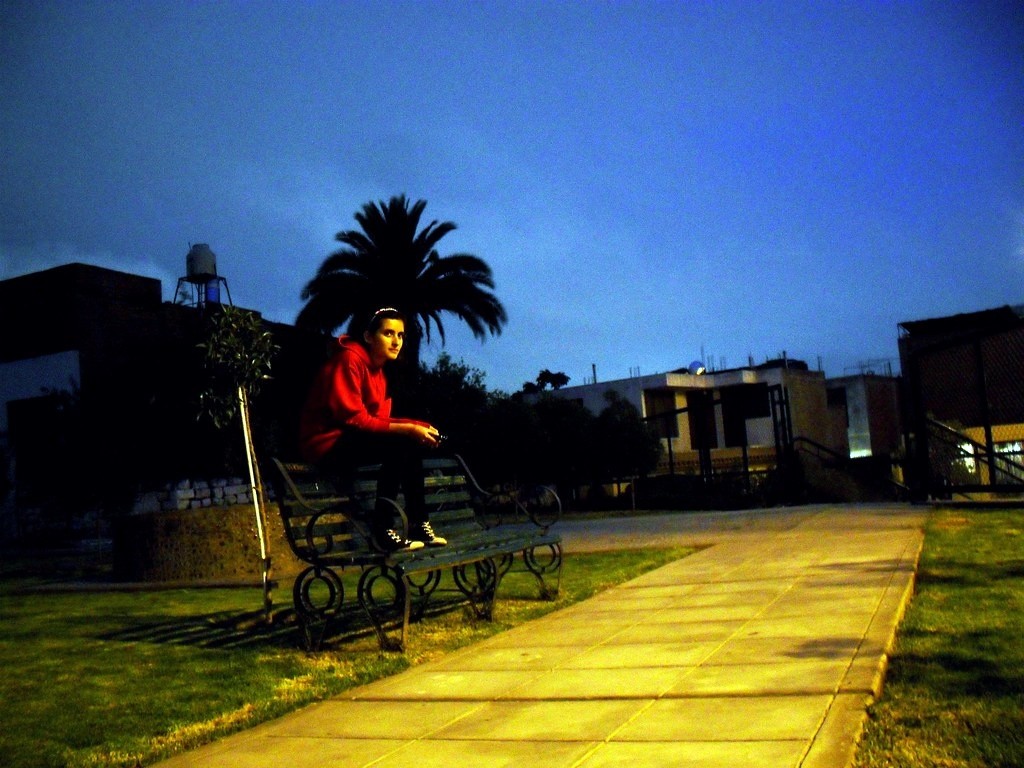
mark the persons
[300,307,447,552]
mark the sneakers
[369,529,424,553]
[407,520,448,546]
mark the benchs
[268,456,565,652]
